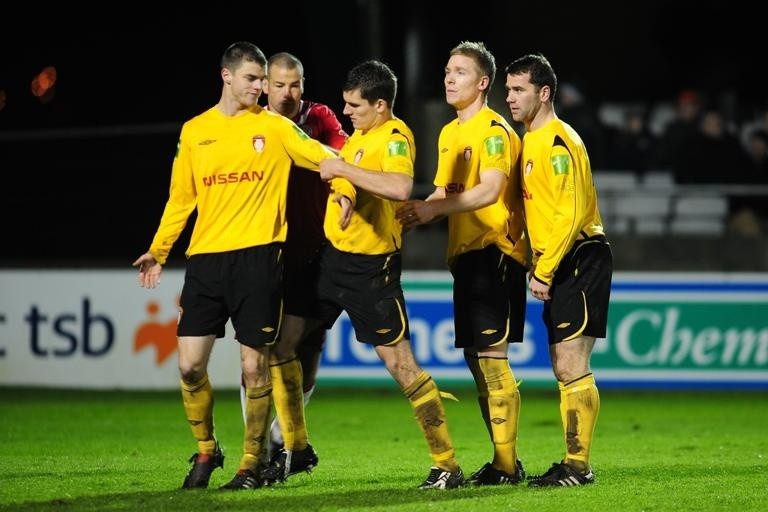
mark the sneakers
[182,448,223,488]
[418,466,466,490]
[225,427,318,489]
[463,460,595,488]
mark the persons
[240,51,350,471]
[557,78,768,184]
[504,54,613,489]
[133,41,357,490]
[394,42,533,487]
[259,59,465,492]
